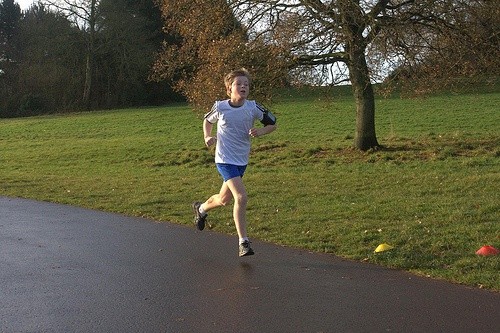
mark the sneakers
[238,241,254,257]
[192,201,208,230]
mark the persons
[192,67,277,257]
